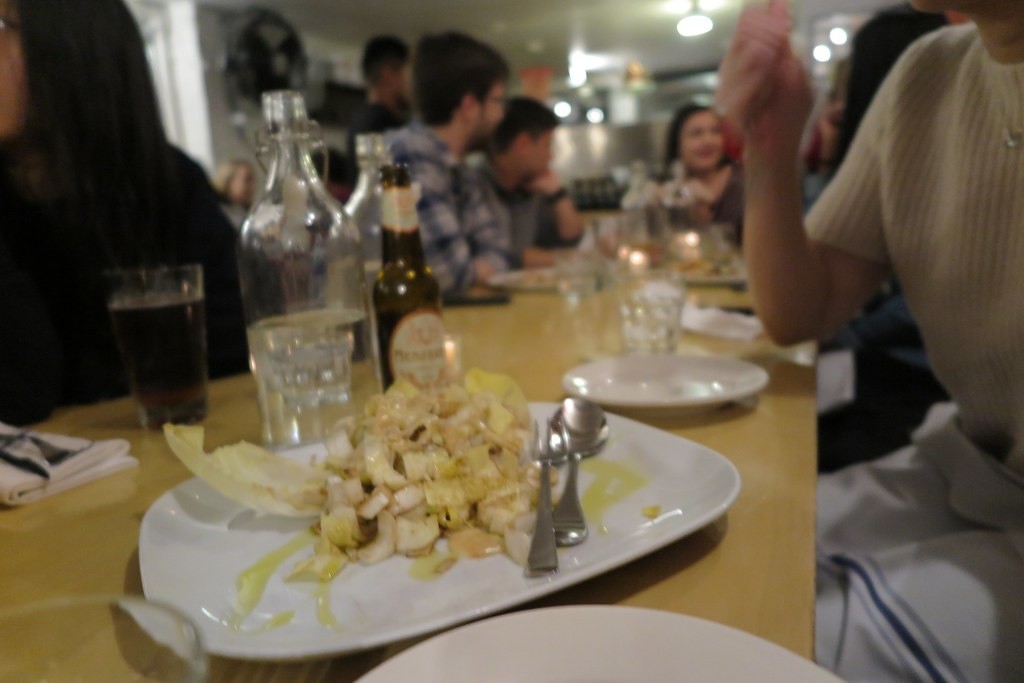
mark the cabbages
[163,368,560,629]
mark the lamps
[676,2,715,37]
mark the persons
[213,11,586,304]
[1,0,250,427]
[646,1,1024,682]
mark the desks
[0,223,818,683]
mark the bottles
[236,92,391,450]
[369,165,444,390]
[331,132,401,333]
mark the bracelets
[544,188,569,205]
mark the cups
[552,249,601,313]
[104,263,212,431]
[617,267,687,352]
[242,312,355,443]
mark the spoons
[549,395,610,546]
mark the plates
[135,399,742,665]
[351,603,846,683]
[685,258,747,285]
[561,352,770,418]
[484,266,555,292]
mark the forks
[528,414,574,575]
